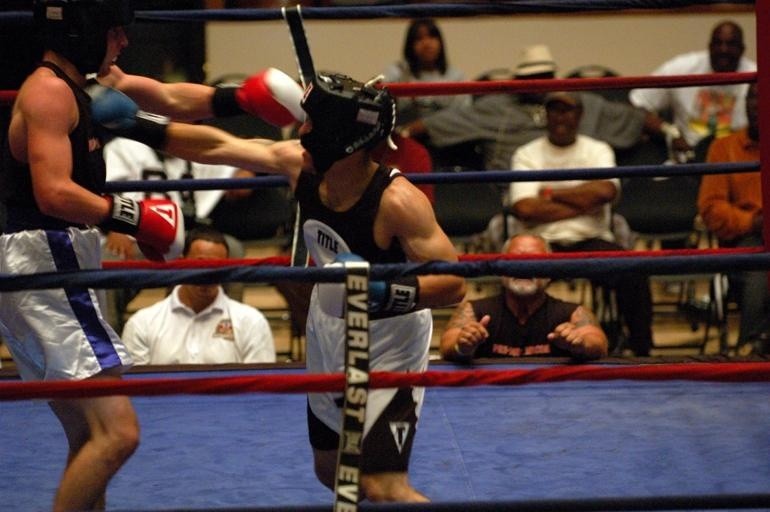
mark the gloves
[232,64,310,132]
[100,192,187,264]
[89,87,170,152]
[316,253,420,319]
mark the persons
[379,22,494,173]
[0,4,306,512]
[626,19,756,159]
[511,90,656,359]
[86,73,432,506]
[162,155,261,207]
[413,44,685,166]
[442,235,610,361]
[699,81,769,359]
[191,70,266,197]
[96,131,184,242]
[121,231,274,365]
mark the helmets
[299,70,398,178]
[48,1,135,77]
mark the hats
[545,92,585,110]
[511,42,557,76]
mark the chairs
[618,178,713,355]
[435,167,504,266]
